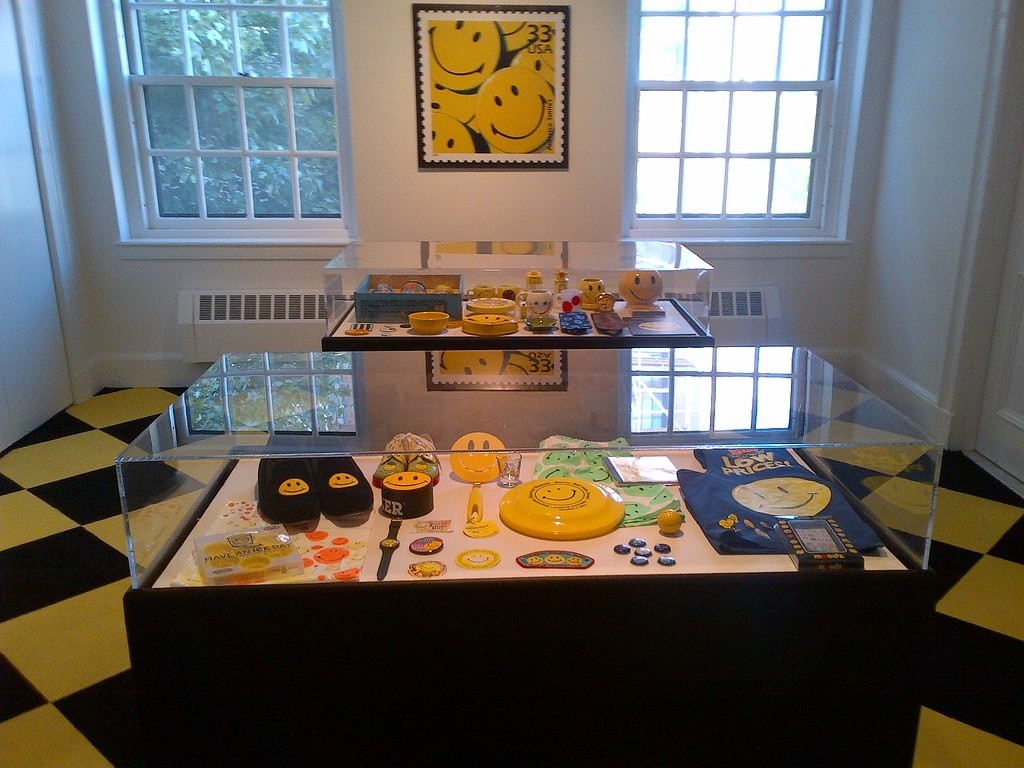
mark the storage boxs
[354,274,462,322]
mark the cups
[496,453,522,488]
[498,285,526,301]
[556,289,583,313]
[516,289,554,318]
[467,284,496,301]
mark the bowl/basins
[408,312,449,334]
